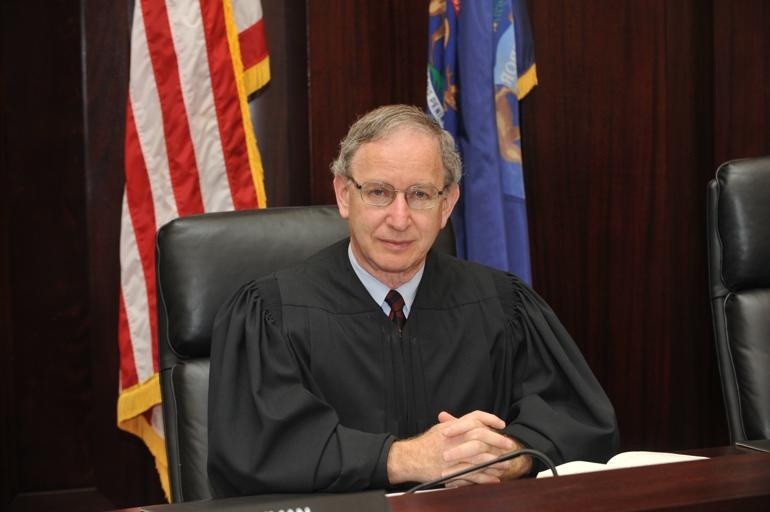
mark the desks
[110,440,768,512]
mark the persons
[201,99,623,500]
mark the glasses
[345,174,448,211]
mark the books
[534,451,712,480]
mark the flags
[420,1,542,296]
[116,2,281,507]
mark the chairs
[152,202,457,506]
[704,154,770,445]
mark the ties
[384,290,408,329]
